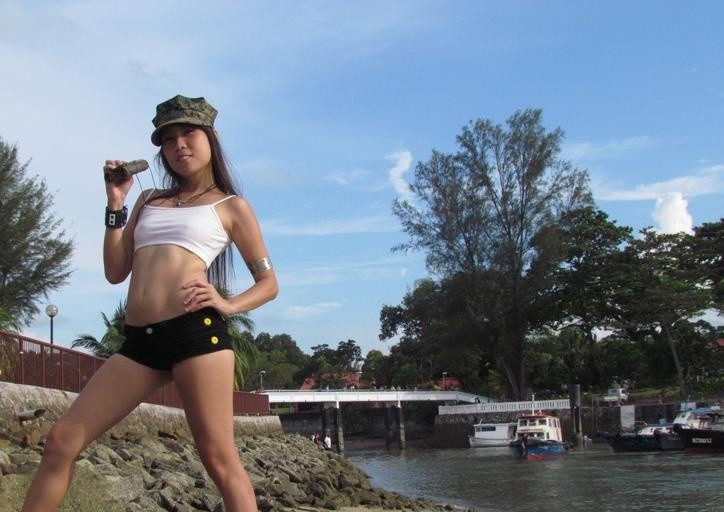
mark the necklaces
[175,184,216,207]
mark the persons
[311,432,332,451]
[21,94,279,512]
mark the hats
[147,94,219,148]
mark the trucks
[591,388,631,406]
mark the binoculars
[104,159,149,182]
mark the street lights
[442,371,448,389]
[44,304,59,366]
[258,370,266,389]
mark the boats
[467,394,566,460]
[596,403,724,454]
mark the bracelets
[105,206,128,228]
